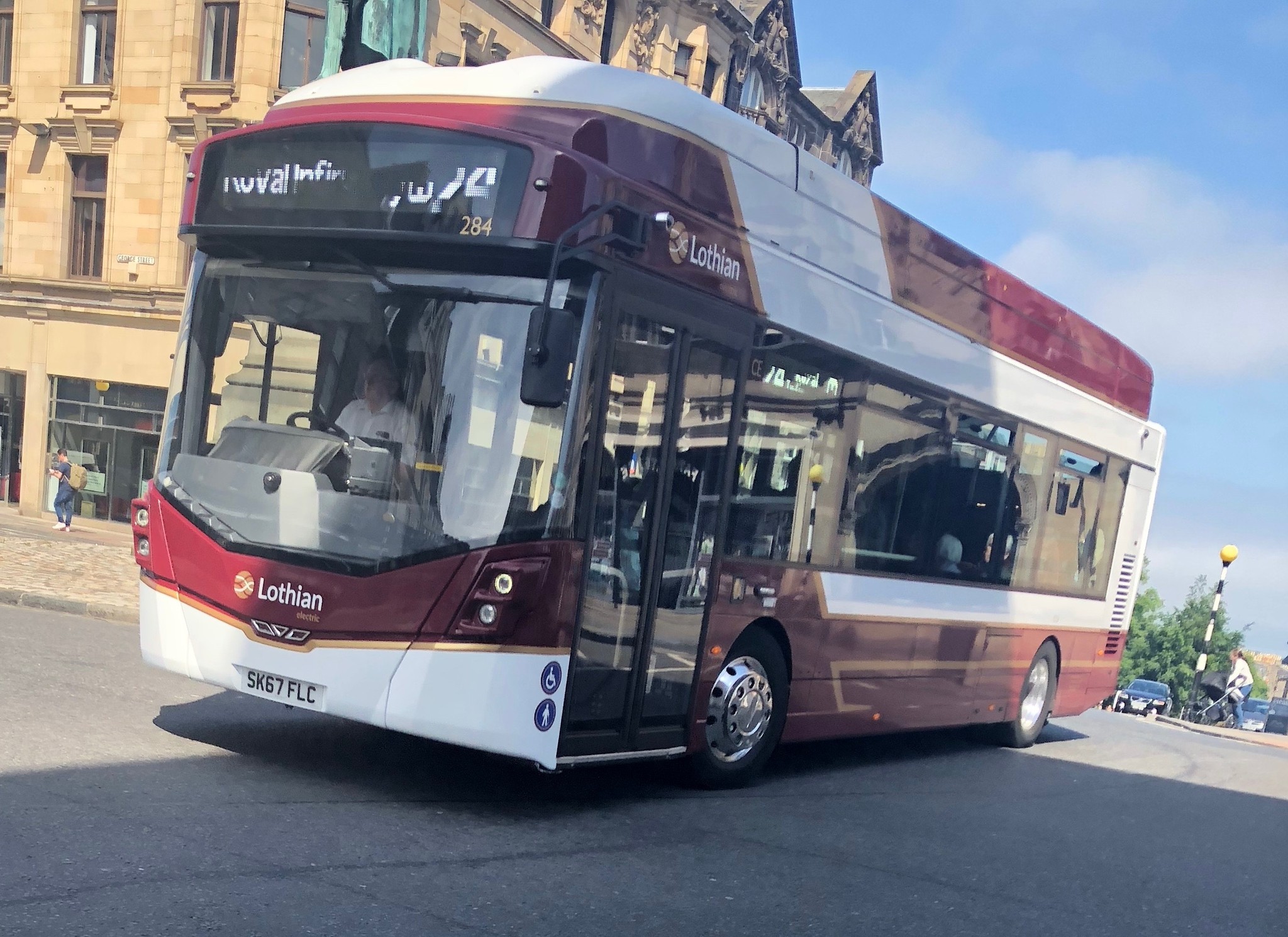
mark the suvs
[1116,678,1173,718]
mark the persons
[929,534,962,578]
[48,448,75,532]
[1095,700,1125,713]
[1151,707,1157,714]
[328,359,420,479]
[979,532,1013,583]
[1075,522,1104,586]
[1225,649,1254,729]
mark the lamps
[436,51,462,67]
[18,119,52,137]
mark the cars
[1238,697,1271,733]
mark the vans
[48,447,105,519]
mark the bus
[127,56,1166,785]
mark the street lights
[806,464,825,564]
[96,381,110,462]
[1182,545,1240,721]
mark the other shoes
[1231,725,1242,731]
[66,527,69,532]
[52,522,66,529]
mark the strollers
[1183,670,1247,725]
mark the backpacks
[63,461,88,491]
